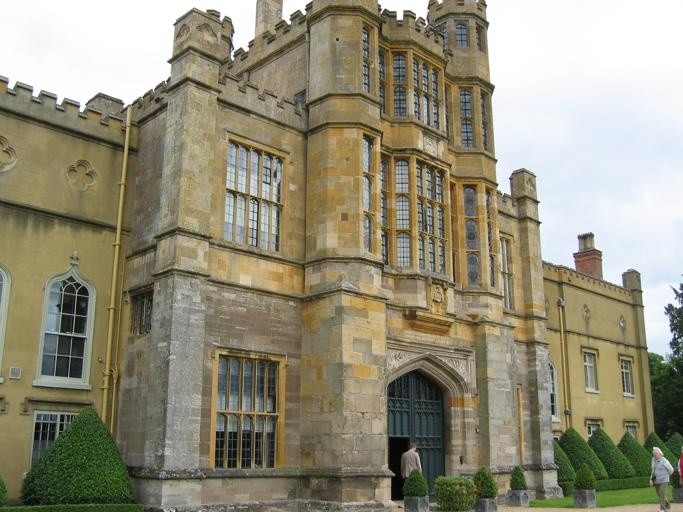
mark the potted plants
[402,461,530,512]
[573,461,599,508]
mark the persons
[678,446,683,485]
[401,443,422,480]
[649,446,674,512]
